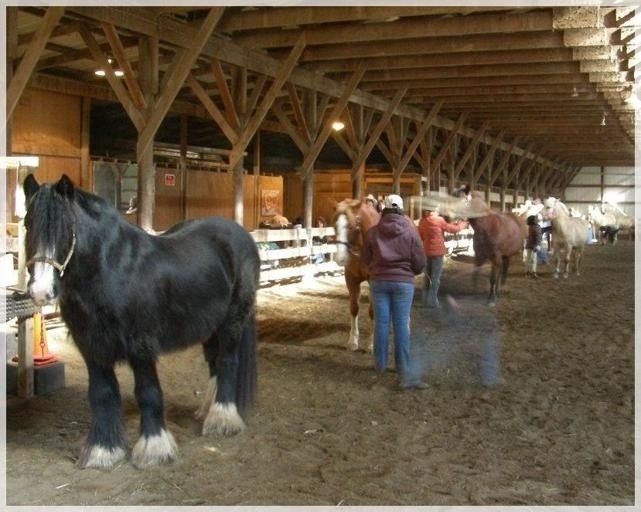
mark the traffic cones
[12,304,58,365]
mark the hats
[363,194,404,209]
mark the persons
[525,215,543,279]
[377,199,386,215]
[544,205,553,253]
[418,209,470,308]
[254,215,332,288]
[363,193,379,211]
[360,193,430,390]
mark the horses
[327,196,382,353]
[535,196,588,279]
[443,182,527,308]
[22,172,262,472]
[591,201,635,246]
